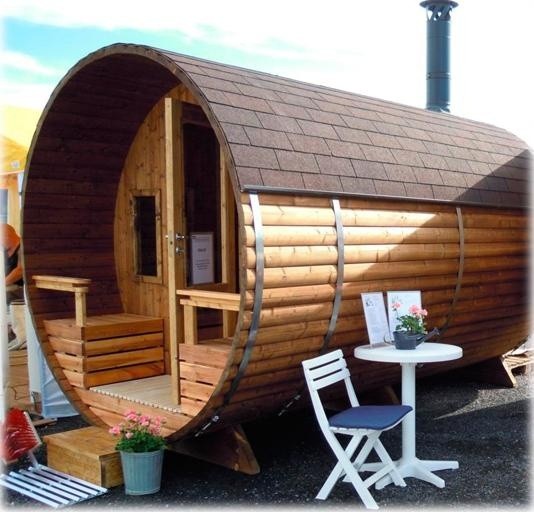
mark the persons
[0,223,27,350]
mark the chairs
[300,348,414,510]
[32,275,168,392]
[173,282,245,420]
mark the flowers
[109,410,166,454]
[391,304,429,332]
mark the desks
[351,342,465,491]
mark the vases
[118,445,164,498]
